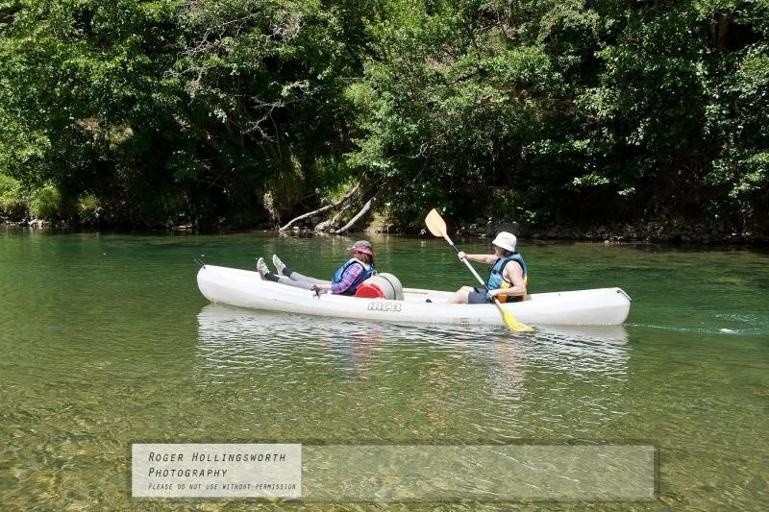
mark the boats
[195,259,633,328]
[192,299,632,363]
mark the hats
[491,230,518,253]
[350,240,373,257]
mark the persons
[257,239,376,296]
[426,231,527,305]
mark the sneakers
[272,254,288,276]
[256,256,271,279]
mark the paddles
[424,208,535,333]
